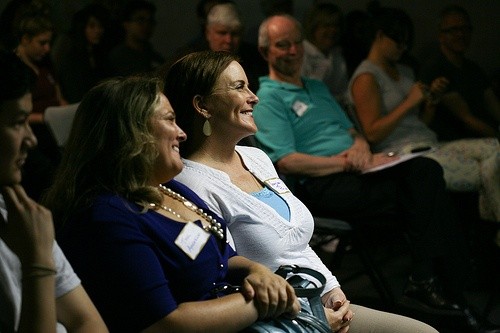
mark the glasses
[438,24,474,33]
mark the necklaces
[151,184,223,237]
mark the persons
[58,0,178,104]
[249,14,473,313]
[180,0,500,247]
[12,16,69,120]
[51,78,301,333]
[0,48,109,332]
[162,51,440,333]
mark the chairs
[248,135,385,294]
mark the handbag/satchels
[214,265,332,333]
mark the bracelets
[20,263,56,280]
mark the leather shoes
[439,305,500,333]
[400,274,462,316]
